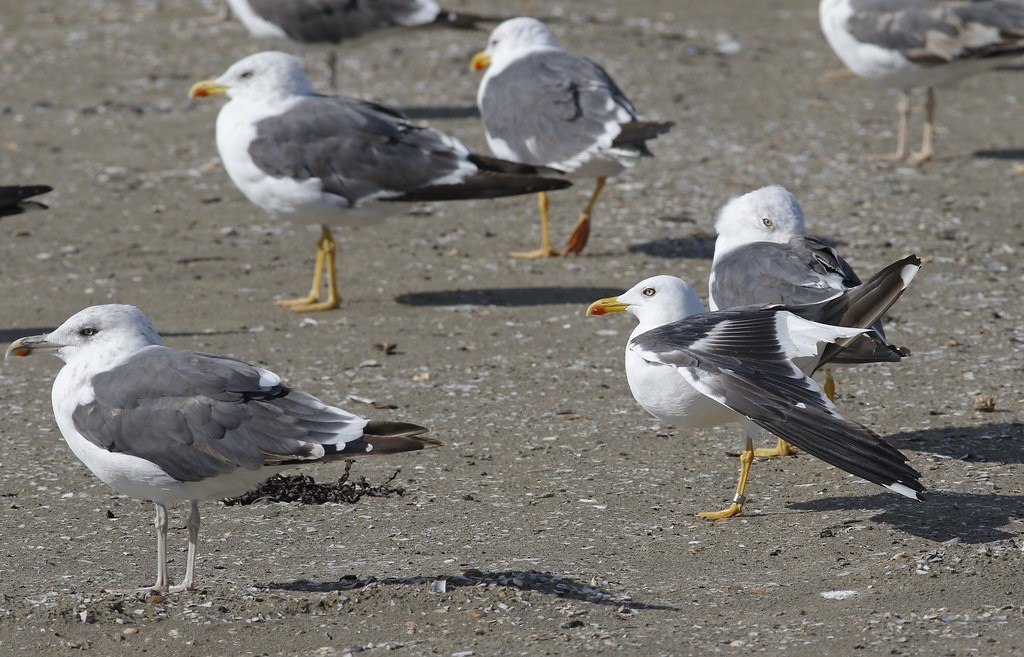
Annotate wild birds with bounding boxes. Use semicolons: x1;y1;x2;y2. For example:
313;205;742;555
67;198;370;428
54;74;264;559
817;1;1024;166
707;184;913;455
470;18;677;257
1;303;443;595
190;50;571;311
585;255;927;521
222;1;480;86
